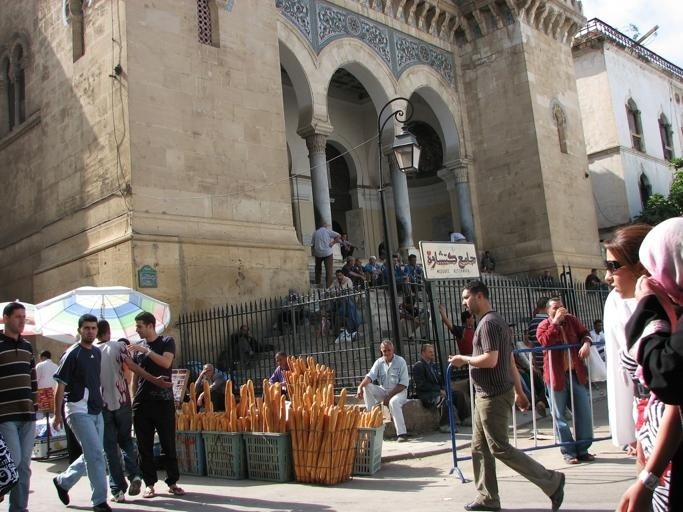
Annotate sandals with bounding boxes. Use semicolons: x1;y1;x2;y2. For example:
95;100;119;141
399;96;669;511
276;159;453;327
168;486;186;496
143;489;155;498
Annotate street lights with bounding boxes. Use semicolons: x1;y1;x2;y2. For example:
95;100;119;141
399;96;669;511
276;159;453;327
378;95;423;357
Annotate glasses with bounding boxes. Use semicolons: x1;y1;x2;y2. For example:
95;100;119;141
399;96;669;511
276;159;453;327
604;260;624;272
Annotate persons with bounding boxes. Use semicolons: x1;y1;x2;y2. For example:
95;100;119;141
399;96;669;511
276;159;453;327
195;223;459;443
440;217;682;512
1;303;184;510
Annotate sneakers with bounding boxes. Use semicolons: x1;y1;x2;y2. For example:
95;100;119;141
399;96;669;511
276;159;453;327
549;473;566;511
397;434;408;443
463;502;501;511
110;490;126;503
52;476;71;506
93;502;112;511
127;478;142;496
537;402;548;418
567;453;595;465
460;419;472;427
439;425;459;433
